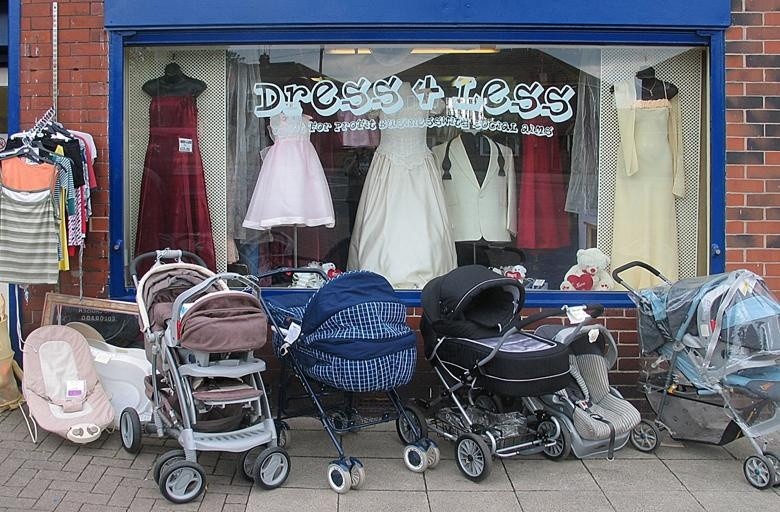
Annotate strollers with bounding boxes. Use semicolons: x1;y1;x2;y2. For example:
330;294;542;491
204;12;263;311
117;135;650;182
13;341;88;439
122;247;292;502
529;318;662;461
611;260;780;489
251;265;439;492
398;261;570;483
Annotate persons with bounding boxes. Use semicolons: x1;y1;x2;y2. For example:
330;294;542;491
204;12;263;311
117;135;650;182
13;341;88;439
133;61;215;277
243;108;335;232
611;70;684;287
345;81;457;290
431;131;520;252
516;92;568;250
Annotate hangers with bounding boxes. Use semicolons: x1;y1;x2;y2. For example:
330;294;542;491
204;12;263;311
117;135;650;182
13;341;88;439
0;110;75;173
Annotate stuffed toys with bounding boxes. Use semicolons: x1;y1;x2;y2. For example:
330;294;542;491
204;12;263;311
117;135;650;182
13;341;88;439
321;262;342;280
562;247;611;294
503;265;526;283
